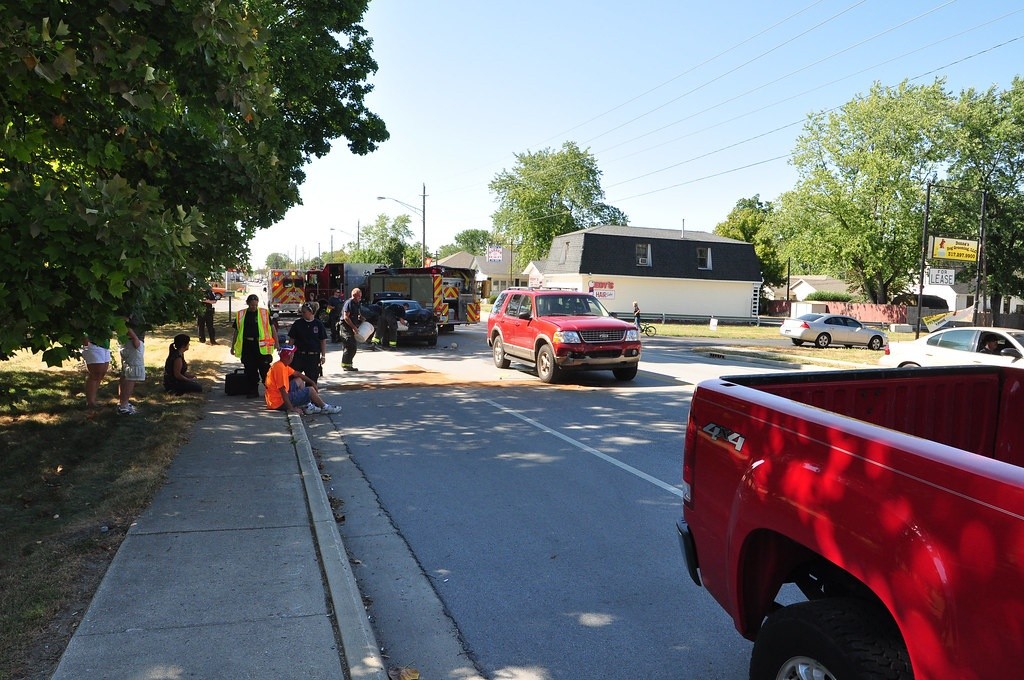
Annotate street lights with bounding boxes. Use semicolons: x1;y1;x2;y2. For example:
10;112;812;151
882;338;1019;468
330;227;360;251
376;196;425;267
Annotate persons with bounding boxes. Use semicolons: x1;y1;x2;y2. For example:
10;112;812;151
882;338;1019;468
230;295;279;398
265;345;342;414
195;284;219;345
163;334;202;393
633;302;642;332
79;332;112;409
117;314;146;415
328;289;342;343
340;288;365;371
371;302;409;351
288;302;328;388
978;333;1001;354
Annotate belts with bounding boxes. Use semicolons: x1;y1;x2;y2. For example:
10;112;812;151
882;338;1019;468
298;350;318;355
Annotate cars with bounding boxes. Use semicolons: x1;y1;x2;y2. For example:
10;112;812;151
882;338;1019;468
878;326;1024;370
369;299;439;347
780;313;887;351
206;281;226;300
247;278;268;292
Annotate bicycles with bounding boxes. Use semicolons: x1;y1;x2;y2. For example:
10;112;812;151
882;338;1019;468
632;315;656;337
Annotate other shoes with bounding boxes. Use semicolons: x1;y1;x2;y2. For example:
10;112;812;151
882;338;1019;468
247;390;259;398
344;365;358;372
116;402;140;415
371;342;376;351
333;338;337;343
338;338;342;342
210;340;218;344
389;346;398;351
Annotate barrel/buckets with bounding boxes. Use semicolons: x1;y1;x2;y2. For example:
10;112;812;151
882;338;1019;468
388;320;409;330
354;322;375;342
710;318;718;330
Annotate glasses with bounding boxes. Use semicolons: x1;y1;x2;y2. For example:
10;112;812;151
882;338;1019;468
335;292;340;293
304;302;314;314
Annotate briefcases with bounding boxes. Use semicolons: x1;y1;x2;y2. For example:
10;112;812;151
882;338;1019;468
225;368;247;395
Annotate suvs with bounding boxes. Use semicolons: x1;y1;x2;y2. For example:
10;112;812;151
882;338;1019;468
487;287;641;382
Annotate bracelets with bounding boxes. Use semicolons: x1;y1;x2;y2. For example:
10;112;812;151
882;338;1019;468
322;356;325;358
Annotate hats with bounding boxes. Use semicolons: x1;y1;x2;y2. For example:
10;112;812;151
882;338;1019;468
278;344;296;357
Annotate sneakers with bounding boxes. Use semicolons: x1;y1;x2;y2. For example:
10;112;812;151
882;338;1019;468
320;404;342;414
305;404;321;415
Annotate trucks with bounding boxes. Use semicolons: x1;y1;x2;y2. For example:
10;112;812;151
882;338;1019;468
263;270;308;316
303;263;483;335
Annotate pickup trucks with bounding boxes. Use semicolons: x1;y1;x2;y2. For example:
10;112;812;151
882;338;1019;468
675;366;1024;680
